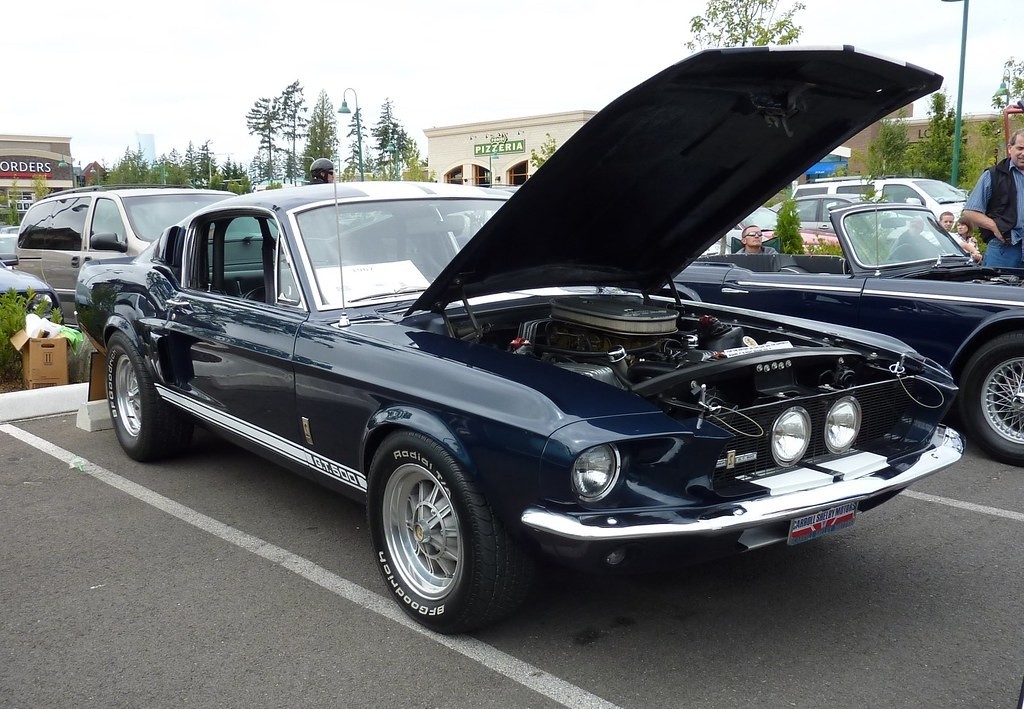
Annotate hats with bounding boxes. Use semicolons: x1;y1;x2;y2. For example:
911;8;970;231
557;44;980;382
909;216;924;230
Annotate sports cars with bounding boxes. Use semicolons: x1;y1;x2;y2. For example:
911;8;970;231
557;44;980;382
618;203;1024;469
72;43;970;639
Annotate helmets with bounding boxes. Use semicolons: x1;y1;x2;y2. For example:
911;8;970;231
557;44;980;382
310;158;333;177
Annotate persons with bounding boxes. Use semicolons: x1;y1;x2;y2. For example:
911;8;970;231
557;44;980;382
735;225;779;254
888;211;983;264
308;158;335;186
963;128;1024;269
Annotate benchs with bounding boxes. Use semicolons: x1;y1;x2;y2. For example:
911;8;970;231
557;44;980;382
208;265;340;304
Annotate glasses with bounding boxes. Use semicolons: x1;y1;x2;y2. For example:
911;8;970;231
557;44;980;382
744;231;761;238
324;171;333;175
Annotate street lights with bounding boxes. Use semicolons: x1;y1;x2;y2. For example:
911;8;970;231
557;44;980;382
337;88;364;181
993;68;1011;161
387;138;400;180
488;143;500;188
58;150;75;188
941;0;970;187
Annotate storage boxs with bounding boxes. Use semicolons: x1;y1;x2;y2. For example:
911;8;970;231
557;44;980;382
10;313;84;390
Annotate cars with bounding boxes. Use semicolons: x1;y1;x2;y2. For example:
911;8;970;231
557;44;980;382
0;174;969;327
